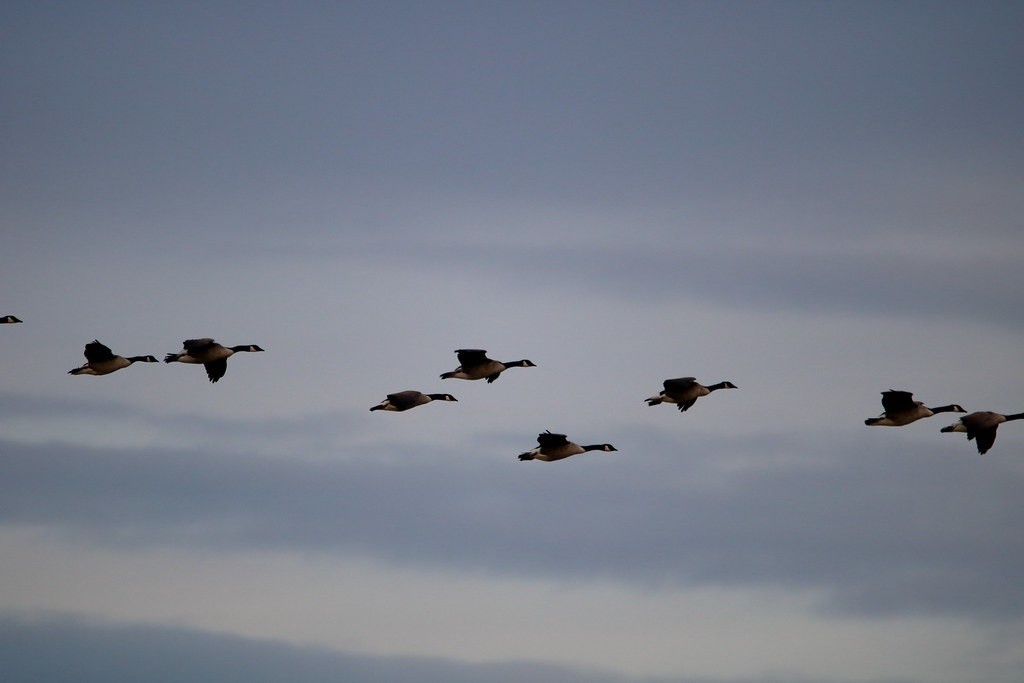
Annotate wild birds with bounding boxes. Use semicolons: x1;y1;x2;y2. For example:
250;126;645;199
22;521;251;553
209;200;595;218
864;389;968;427
644;377;738;412
940;411;1024;455
439;349;537;383
517;430;619;462
66;339;161;376
163;338;265;384
367;390;458;412
